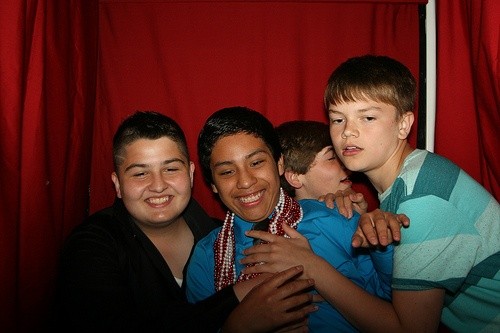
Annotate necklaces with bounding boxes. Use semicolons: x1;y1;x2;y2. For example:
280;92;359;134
214;186;303;293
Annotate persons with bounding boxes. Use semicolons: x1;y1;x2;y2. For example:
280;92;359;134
53;110;319;333
270;118;353;200
237;54;500;333
181;105;411;333
